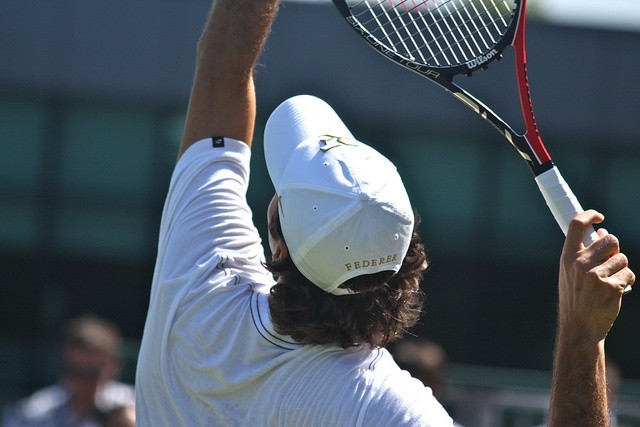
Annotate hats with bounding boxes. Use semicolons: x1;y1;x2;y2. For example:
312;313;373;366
263;95;415;296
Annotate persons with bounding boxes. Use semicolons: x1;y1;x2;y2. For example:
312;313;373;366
0;312;136;427
135;0;636;427
